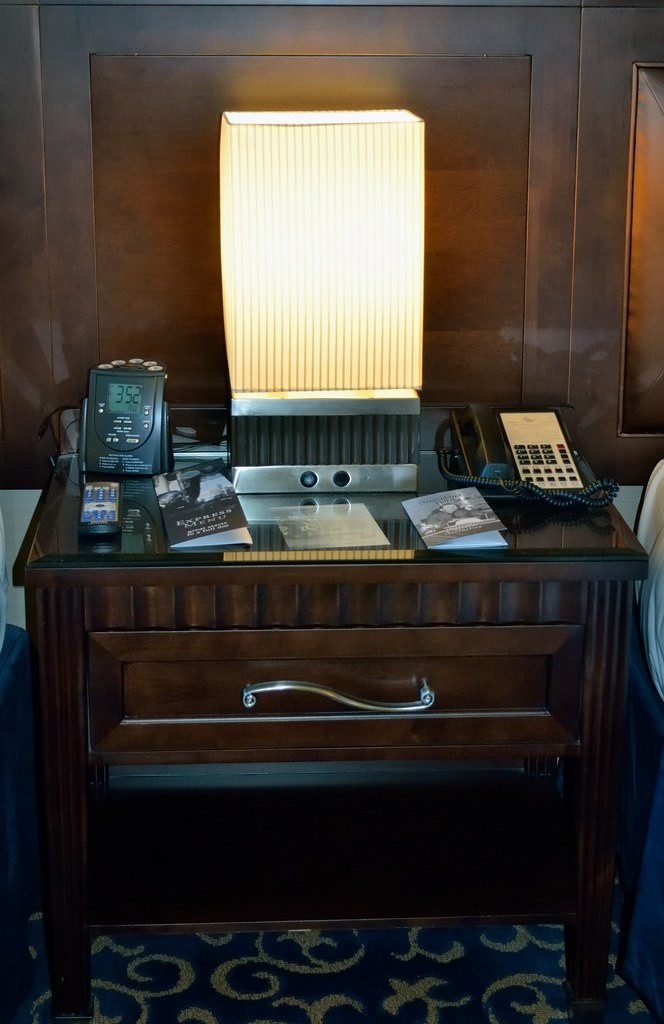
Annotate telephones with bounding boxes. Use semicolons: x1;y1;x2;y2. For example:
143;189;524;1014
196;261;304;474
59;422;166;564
452;405;606;508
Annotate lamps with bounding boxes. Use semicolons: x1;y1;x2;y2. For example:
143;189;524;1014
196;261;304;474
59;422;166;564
220;109;425;493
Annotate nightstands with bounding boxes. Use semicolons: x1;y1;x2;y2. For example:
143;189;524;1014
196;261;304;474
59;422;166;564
12;456;649;1024
